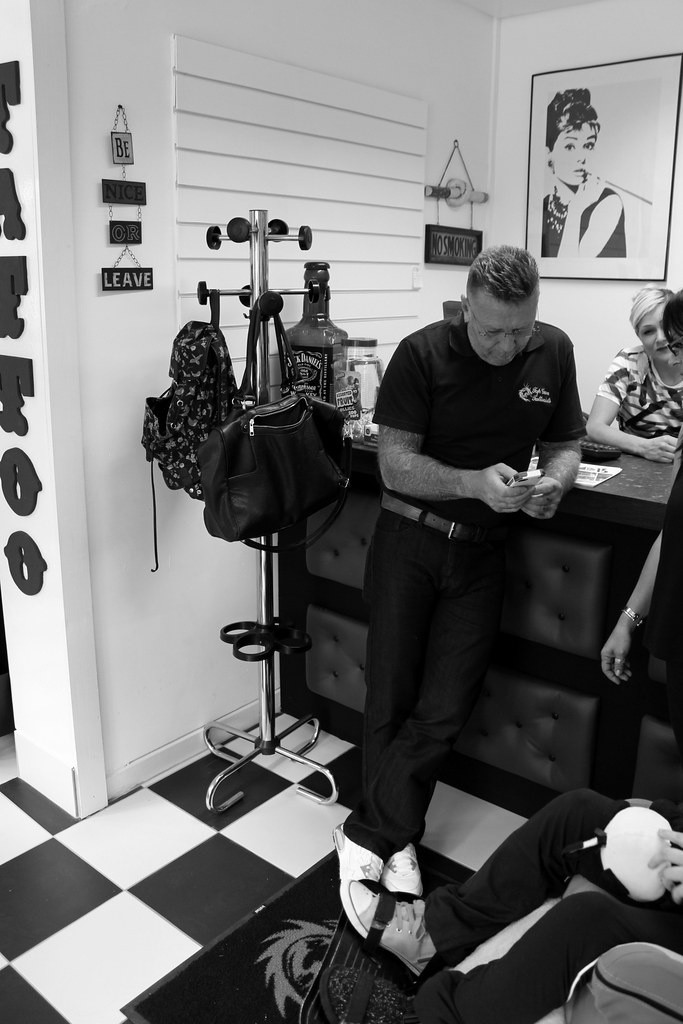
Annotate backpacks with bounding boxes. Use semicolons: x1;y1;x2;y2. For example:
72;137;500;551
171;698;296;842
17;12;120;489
141;289;238;501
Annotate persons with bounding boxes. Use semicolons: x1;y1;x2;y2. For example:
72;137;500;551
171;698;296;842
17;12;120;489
321;787;683;1024
332;247;588;896
603;290;683;749
586;283;683;462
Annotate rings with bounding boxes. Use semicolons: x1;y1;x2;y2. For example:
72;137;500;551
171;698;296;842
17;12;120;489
615;658;625;663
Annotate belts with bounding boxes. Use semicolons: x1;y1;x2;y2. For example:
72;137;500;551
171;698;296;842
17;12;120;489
380;491;489;543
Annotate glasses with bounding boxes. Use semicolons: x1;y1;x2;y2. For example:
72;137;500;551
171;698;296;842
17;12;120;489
667;336;683;356
467;300;541;341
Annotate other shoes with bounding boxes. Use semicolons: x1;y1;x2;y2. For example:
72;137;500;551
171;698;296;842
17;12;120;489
318;965;418;1024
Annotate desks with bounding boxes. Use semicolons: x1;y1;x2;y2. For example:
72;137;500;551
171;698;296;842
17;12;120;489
278;454;683;812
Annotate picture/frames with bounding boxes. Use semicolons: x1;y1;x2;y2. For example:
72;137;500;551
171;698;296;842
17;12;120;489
528;53;683;282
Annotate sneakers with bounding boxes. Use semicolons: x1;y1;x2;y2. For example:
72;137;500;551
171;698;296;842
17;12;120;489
333;823;383;883
381;842;424;896
338;878;437;977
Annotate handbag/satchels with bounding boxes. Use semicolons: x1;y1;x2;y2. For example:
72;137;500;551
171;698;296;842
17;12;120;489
196;297;351;553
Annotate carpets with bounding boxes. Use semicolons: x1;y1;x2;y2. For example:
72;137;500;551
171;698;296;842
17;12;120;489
121;844;477;1024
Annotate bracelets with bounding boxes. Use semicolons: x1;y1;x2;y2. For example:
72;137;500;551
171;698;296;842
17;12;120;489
621;606;643;627
